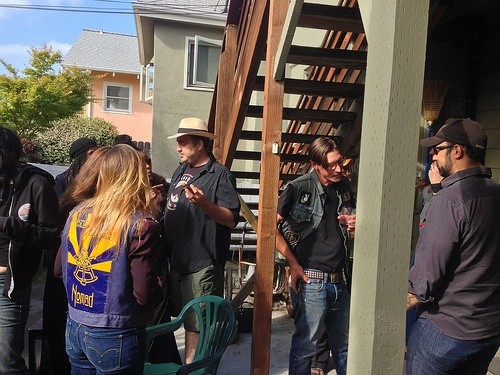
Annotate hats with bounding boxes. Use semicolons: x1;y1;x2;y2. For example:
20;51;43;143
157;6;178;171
0;127;24;167
341;154;351;166
69;137;98;158
420;118;488;149
167;117;217;141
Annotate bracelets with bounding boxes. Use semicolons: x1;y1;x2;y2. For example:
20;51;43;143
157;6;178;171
430;183;444;193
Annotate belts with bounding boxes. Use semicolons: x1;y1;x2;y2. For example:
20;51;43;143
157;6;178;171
303;270;343;283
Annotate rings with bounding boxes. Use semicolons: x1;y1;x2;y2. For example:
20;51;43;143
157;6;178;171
430;169;431;170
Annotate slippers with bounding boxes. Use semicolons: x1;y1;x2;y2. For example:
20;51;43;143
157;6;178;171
311;368;325;375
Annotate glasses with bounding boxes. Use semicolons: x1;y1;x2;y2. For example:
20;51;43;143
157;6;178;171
145;158;152;166
433;145;455;155
327;157;346;170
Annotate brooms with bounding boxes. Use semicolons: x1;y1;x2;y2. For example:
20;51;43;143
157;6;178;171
423;80;448;184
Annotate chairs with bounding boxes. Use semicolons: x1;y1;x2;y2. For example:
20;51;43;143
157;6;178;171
143;295;236;375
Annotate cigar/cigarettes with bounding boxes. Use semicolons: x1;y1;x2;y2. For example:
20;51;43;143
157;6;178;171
181;182;195;196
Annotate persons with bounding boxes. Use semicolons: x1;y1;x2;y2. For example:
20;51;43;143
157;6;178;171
43;135;171;232
418;148;445;233
39;146;113;375
275;136;356;375
164;117;241;364
55;144;166;375
311;159;355;375
0;126;54;375
406;118;500;375
